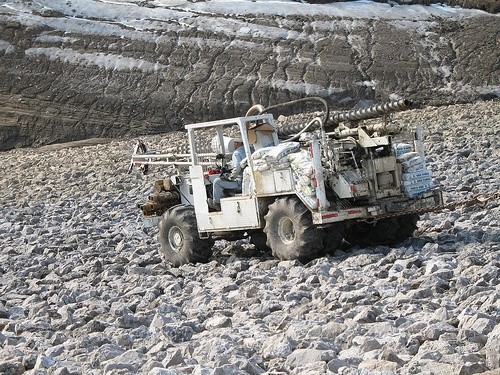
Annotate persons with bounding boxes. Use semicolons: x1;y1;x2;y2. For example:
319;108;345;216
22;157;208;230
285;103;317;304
206;136;253;209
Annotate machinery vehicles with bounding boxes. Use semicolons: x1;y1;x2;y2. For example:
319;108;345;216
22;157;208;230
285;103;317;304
126;114;444;269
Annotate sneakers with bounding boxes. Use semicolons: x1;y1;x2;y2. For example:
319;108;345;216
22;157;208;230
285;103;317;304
208;198;220;210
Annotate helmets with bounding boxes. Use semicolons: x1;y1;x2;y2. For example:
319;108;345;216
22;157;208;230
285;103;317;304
233;132;243;142
